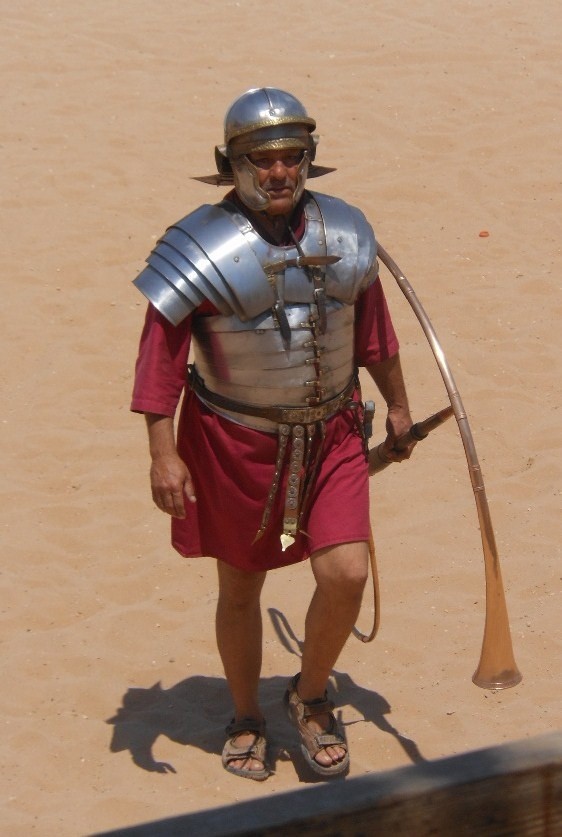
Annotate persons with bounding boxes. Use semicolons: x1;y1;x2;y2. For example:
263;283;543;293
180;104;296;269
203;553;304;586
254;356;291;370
134;85;419;779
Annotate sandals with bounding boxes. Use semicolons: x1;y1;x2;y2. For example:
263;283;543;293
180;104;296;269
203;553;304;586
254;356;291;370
222;717;271;781
283;672;350;776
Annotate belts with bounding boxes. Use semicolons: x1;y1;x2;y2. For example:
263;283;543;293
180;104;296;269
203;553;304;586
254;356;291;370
186;363;359;422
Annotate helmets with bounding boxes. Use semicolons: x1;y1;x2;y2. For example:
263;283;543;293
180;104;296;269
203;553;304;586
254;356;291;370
189;87;338;211
